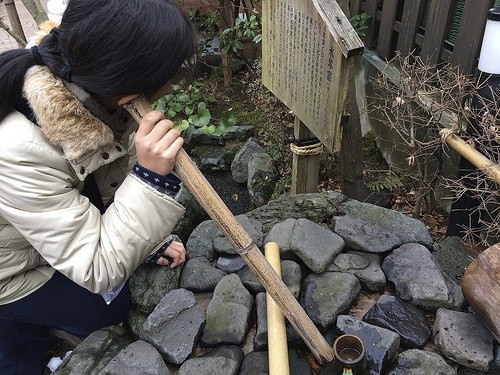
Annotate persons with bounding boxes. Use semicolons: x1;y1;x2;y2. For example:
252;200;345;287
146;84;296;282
0;1;194;347
